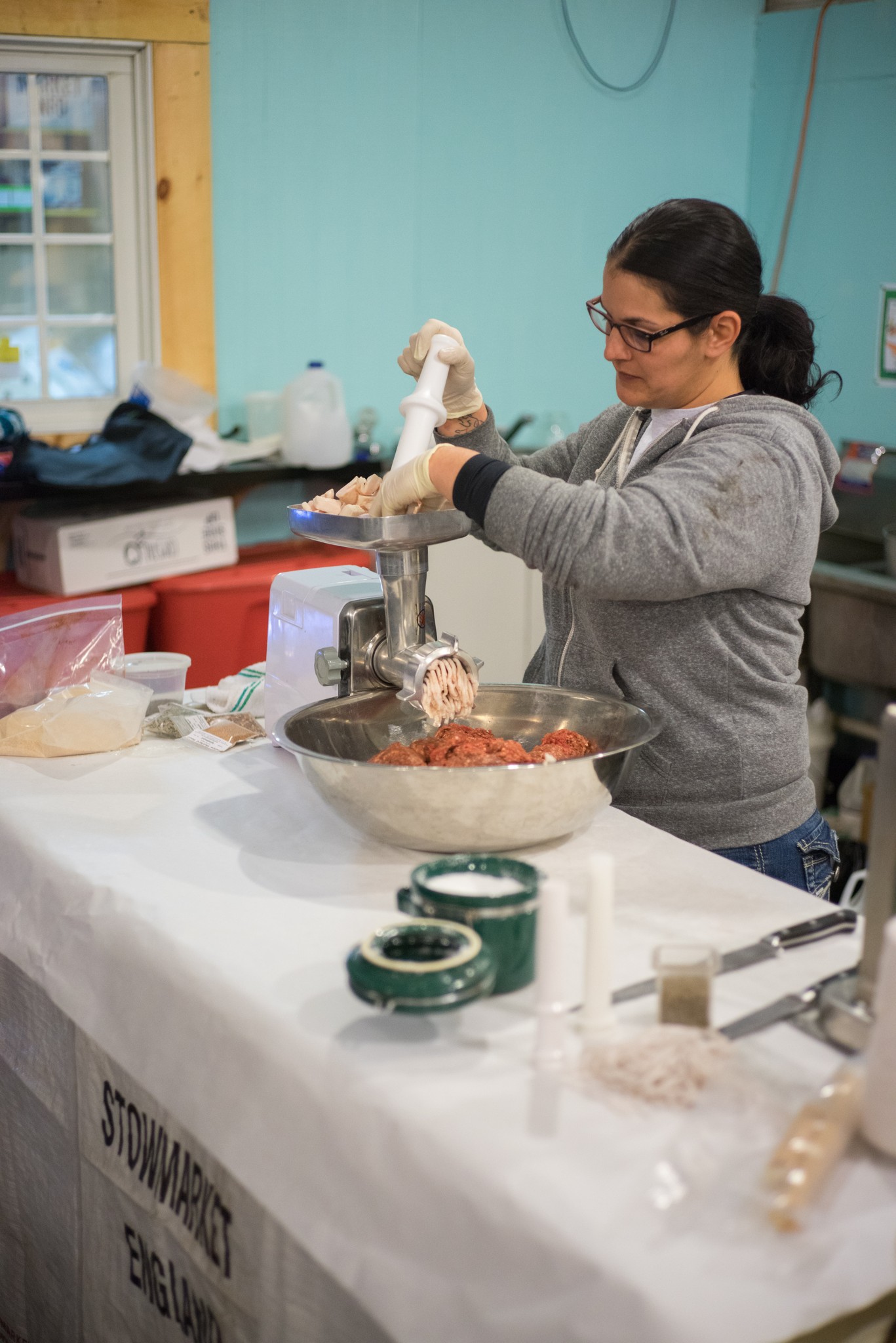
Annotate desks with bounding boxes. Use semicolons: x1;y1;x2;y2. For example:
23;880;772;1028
1;680;894;1342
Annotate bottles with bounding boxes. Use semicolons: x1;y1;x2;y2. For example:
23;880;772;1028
654;935;712;1030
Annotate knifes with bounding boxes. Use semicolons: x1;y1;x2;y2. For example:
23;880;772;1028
718;967;862;1040
564;908;861;1014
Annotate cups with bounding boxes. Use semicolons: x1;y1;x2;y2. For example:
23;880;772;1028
395;858;540;999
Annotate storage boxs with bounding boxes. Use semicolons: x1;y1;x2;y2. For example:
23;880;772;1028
1;490;376;716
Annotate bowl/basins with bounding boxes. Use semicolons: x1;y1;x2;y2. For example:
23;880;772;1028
114;648;191;714
268;677;665;856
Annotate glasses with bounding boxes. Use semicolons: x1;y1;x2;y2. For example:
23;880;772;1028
585;294;719;353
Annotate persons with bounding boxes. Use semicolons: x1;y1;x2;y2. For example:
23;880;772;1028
372;198;842;903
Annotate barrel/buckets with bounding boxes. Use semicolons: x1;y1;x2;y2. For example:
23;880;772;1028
281;357;354;472
